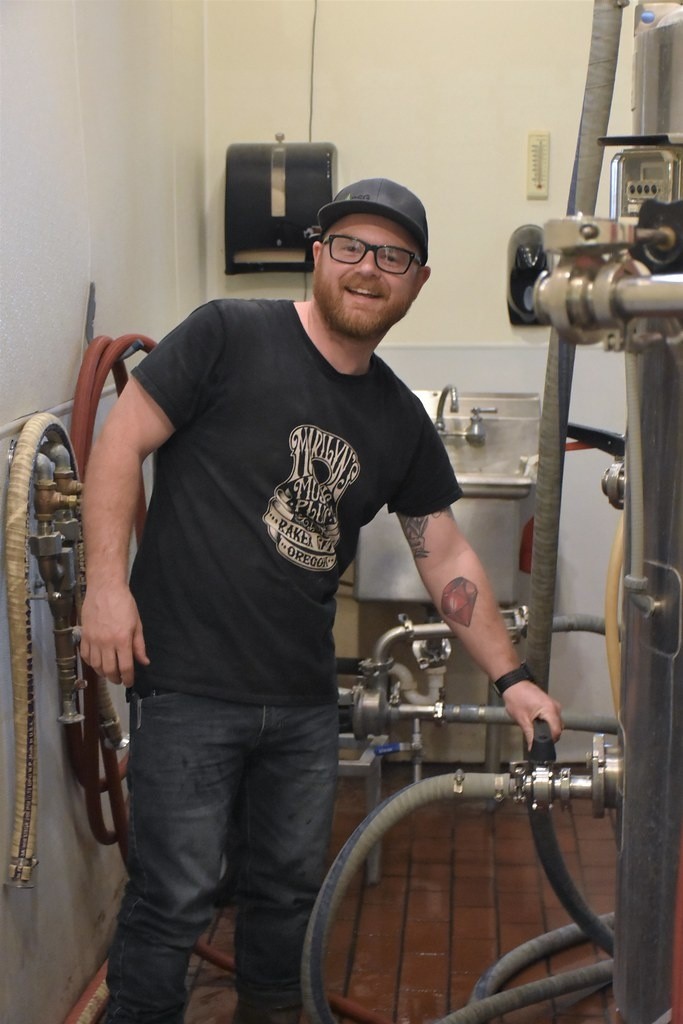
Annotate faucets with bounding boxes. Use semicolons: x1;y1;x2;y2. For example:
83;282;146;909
433;383;499;448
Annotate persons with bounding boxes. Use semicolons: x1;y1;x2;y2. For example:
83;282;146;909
81;178;562;1024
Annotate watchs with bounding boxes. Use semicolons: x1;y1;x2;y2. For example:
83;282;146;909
493;662;534;697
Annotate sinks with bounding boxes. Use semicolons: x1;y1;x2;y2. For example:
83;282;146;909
429;414;541;485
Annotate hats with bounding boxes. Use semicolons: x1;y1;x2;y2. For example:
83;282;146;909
316;178;428;266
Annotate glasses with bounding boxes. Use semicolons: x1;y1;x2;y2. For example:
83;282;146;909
322;234;422;274
